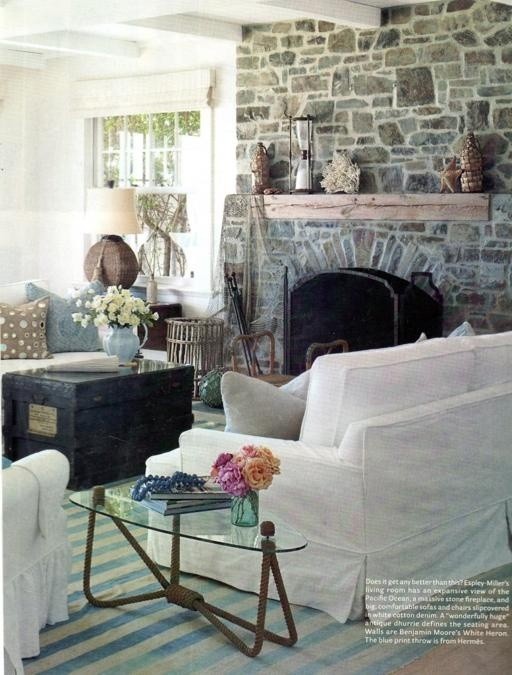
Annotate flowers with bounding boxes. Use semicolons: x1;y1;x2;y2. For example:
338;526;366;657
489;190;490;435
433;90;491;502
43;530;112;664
209;444;284;498
71;283;161;331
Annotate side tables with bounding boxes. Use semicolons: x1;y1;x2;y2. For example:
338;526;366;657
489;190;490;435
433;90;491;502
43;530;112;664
69;473;309;656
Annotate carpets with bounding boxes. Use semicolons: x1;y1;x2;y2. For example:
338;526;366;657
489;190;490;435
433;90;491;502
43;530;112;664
22;402;512;675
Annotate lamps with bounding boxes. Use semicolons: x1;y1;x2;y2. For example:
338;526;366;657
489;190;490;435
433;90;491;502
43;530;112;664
82;180;145;294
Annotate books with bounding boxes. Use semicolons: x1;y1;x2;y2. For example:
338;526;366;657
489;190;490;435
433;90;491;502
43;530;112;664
136;471;233;517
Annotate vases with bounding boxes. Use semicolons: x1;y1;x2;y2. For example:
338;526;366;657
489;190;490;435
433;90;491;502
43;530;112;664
229;493;259;528
146;281;157;303
104;327;149;365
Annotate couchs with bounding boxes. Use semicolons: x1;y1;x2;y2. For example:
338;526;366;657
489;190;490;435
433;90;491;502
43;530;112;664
0;277;125;373
2;447;71;675
146;330;511;623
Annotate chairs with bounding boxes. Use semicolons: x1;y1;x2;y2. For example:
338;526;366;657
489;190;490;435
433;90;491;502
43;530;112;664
306;339;348;371
230;332;297;388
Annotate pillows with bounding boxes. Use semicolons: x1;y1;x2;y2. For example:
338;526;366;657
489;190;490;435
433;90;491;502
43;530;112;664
27;280;102;352
222;372;306;441
0;296;55;358
447;321;477;338
281;370;310;400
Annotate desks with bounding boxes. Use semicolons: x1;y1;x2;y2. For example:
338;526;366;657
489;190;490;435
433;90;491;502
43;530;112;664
137;302;182;350
